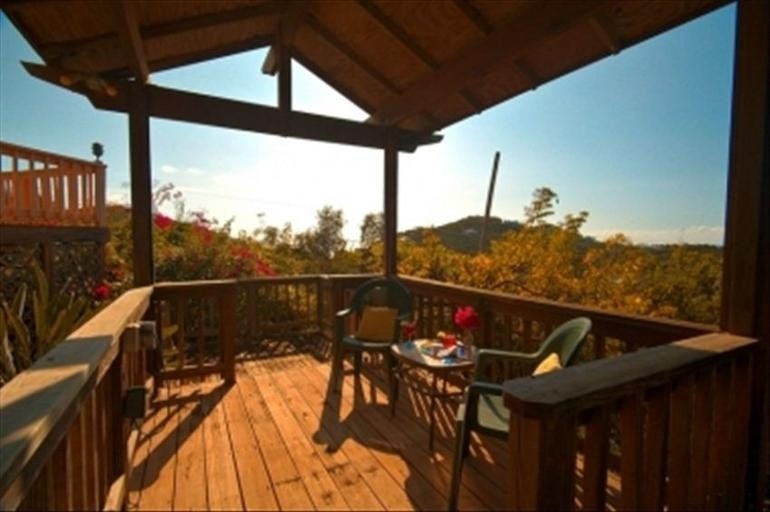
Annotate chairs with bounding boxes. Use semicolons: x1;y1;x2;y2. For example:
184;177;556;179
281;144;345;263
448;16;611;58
334;278;413;413
448;317;593;512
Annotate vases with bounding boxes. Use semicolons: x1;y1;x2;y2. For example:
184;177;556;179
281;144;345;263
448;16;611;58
463;330;473;361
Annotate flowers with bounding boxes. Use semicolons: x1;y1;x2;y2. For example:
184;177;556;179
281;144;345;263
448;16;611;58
453;305;480;330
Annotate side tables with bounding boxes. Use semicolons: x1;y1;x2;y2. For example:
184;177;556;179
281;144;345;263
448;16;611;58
390;339;476;453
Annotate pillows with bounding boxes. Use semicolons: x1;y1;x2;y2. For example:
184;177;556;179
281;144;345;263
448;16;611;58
531;352;562;376
355;305;399;341
